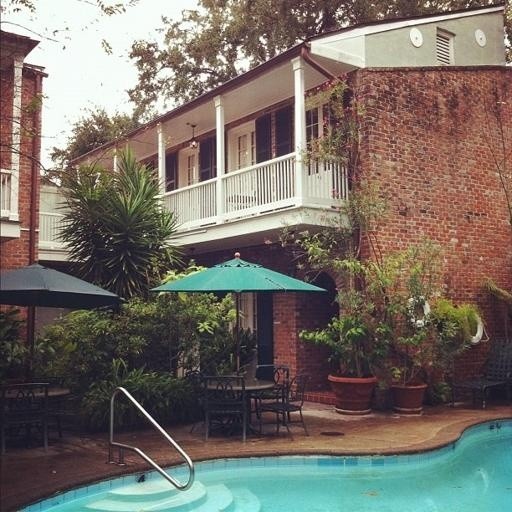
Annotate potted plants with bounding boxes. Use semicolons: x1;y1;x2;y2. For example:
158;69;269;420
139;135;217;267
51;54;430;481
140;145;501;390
296;286;379;416
254;174;463;415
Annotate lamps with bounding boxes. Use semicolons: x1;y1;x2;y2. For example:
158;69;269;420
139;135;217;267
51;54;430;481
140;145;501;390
188;124;199;148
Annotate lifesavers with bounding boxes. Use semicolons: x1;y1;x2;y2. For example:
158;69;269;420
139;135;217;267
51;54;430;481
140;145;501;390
462;311;485;345
407;296;431;328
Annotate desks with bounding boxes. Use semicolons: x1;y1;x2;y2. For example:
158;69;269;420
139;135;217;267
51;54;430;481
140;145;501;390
1;386;72;441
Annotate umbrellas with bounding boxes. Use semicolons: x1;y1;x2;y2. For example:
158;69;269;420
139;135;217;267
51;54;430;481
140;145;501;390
0;258;129;391
149;251;329;386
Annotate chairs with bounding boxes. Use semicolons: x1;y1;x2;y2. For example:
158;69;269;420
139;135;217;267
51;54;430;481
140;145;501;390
444;339;512;412
37;369;65;439
183;361;311;445
1;381;52;454
